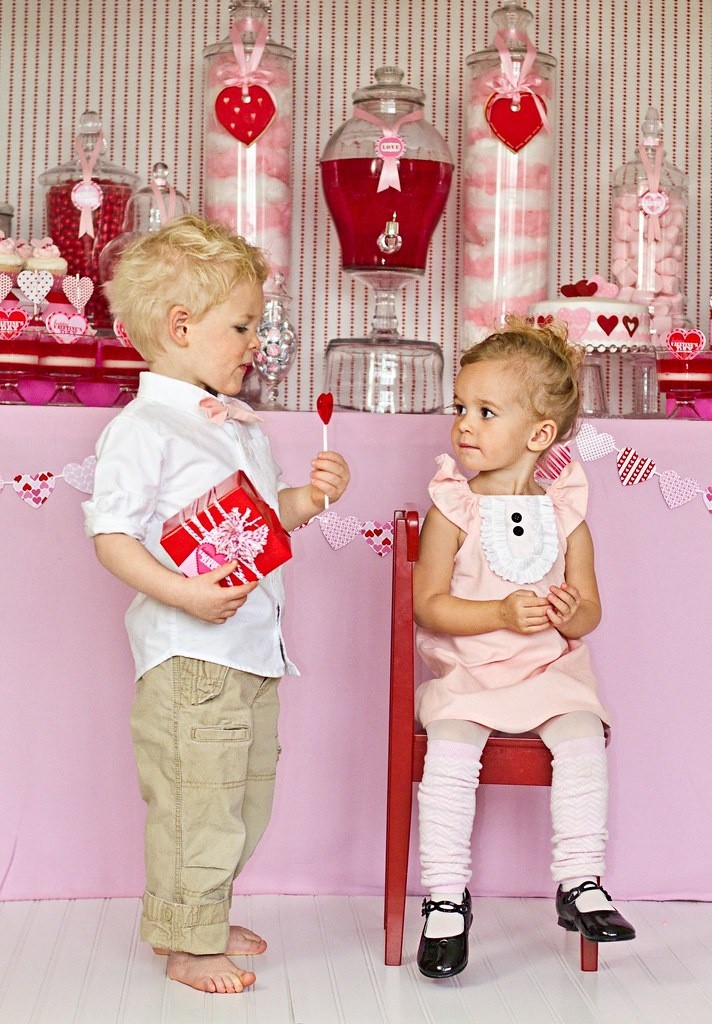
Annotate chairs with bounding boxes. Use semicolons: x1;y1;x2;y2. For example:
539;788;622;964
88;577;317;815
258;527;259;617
383;510;612;972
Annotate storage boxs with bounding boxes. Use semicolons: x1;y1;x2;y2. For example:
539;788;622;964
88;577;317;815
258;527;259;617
160;470;292;587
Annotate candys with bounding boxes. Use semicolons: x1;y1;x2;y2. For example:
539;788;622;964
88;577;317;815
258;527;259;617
317;392;334;425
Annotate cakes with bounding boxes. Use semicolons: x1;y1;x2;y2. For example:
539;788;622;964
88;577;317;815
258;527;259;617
522;273;651;347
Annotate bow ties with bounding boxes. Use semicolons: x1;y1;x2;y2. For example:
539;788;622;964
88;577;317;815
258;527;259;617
201;398;264;427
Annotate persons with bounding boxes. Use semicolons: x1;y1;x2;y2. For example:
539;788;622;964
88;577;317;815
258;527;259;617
409;319;640;980
82;214;354;998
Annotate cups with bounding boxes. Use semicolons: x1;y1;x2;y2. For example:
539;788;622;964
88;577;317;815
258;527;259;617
656;350;712;421
0;331;38;404
98;336;149;406
39;332;98;404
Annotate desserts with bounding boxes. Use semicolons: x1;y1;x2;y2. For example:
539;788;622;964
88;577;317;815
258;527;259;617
0;229;68;277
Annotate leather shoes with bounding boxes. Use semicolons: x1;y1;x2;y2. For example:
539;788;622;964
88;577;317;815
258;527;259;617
417;887;473;979
557;883;636;942
